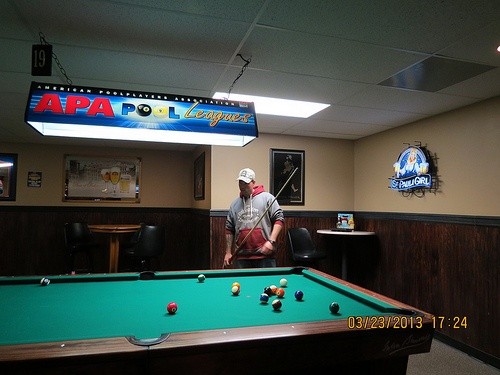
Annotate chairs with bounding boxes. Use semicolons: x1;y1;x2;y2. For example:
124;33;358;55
63;222;96;273
287;227;319;268
125;226;164;272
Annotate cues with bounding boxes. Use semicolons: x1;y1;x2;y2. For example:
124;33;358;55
224;167;298;270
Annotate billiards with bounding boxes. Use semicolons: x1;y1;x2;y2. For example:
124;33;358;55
295;290;303;299
198;274;205;282
280;279;287;287
168;303;177;314
272;300;282;310
329;302;339;313
41;278;50;285
259;285;284;303
232;282;240;295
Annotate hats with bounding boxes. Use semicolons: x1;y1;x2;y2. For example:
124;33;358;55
236;168;255;184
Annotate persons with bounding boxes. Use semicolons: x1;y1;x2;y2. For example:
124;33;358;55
221;168;285;269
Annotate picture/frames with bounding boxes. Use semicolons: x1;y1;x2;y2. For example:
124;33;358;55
193;151;206;200
62;154;143;203
0;152;19;202
269;148;305;206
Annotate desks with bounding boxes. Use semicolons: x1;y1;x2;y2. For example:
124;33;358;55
88;223;142;273
0;267;436;375
317;228;375;281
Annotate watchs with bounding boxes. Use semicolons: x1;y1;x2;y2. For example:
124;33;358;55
268;239;276;245
